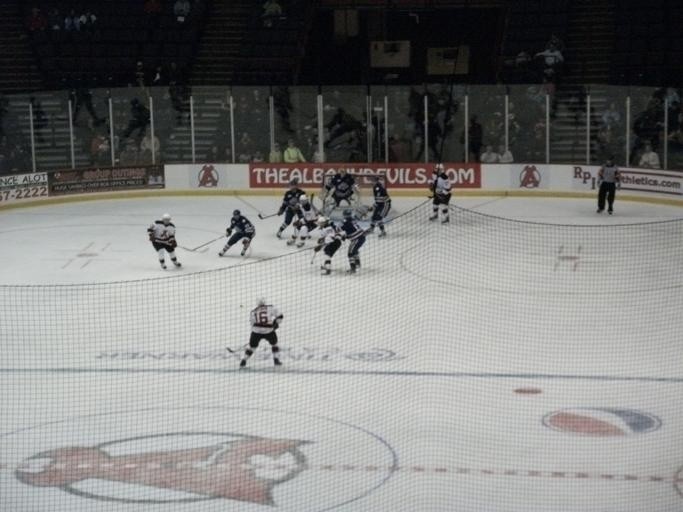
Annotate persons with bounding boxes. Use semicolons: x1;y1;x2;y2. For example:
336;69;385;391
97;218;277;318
428;161;452;224
593;154;622;216
275;166;392;277
0;0;683;171
239;295;284;370
215;208;255;258
144;213;182;271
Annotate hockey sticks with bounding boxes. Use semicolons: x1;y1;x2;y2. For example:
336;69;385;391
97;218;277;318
156;235;226;253
258;212;276;219
226;343;248;353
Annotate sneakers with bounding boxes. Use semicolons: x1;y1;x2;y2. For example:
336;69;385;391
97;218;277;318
609;211;612;215
240;359;247;366
161;264;167;270
430;214;438;220
241;249;245;256
275;358;281;365
378;233;386;237
366;227;374;232
172;261;181;266
596;209;603;213
218;249;224;256
442;218;449;223
321;259;332;273
346;258;360;272
277;229;304;247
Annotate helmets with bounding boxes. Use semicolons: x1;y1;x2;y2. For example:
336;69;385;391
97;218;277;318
162;213;170;218
233;210;240;215
256;300;264;306
437;165;444;169
290;168;353;224
